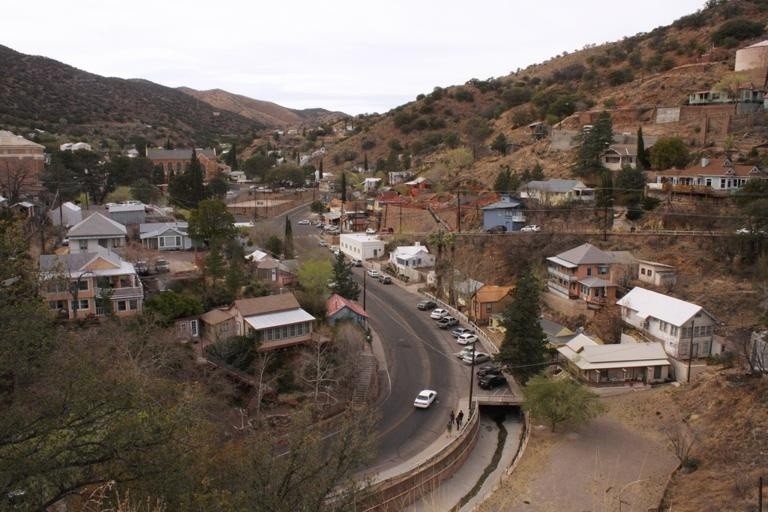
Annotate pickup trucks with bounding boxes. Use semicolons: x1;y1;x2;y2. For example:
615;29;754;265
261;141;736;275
437;317;460;329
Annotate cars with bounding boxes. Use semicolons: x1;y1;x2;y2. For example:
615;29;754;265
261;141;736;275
297;220;345;257
457;346;475;359
463;352;491;365
155;259;170;272
103;201;154;212
477;366;497;376
248;185;309;194
134;260;149;273
736;227;766;235
431;308;449;319
367;270;378;278
414;389;437;408
366;228;376;235
451;328;475;336
417;300;436;310
479;375;507;389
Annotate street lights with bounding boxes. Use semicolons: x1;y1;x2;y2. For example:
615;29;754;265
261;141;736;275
604;197;615;240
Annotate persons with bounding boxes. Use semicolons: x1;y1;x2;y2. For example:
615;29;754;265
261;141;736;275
450;410;454;424
459;409;464;426
456;415;461;430
446;421;452;438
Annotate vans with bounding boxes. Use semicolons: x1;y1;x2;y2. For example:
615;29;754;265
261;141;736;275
378;274;391;284
379;227;394;235
350;258;362;266
520;225;542;232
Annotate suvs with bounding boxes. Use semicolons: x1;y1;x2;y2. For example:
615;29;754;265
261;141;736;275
487;224;507;234
457;334;478;345
